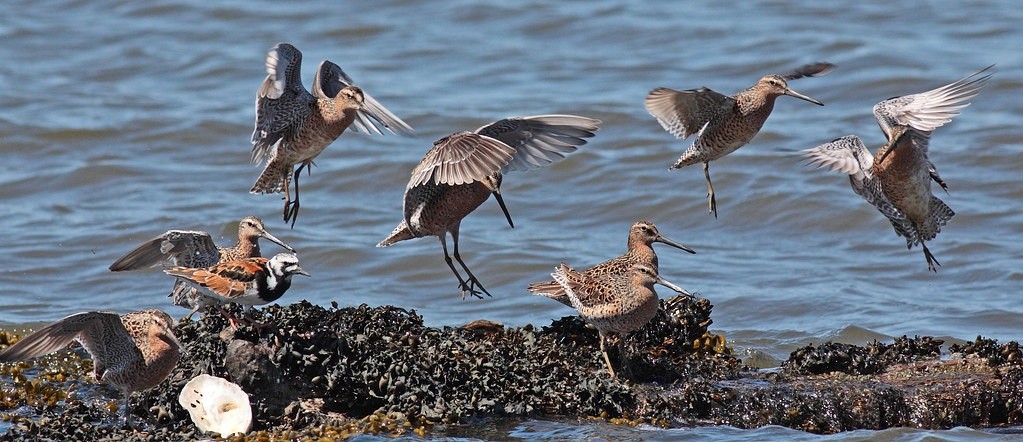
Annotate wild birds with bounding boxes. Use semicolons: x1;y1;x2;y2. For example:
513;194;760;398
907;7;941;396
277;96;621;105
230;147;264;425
108;215;312;334
643;61;839;221
792;62;1000;274
526;219;698;383
249;42;419;231
374;113;603;301
0;308;189;434
